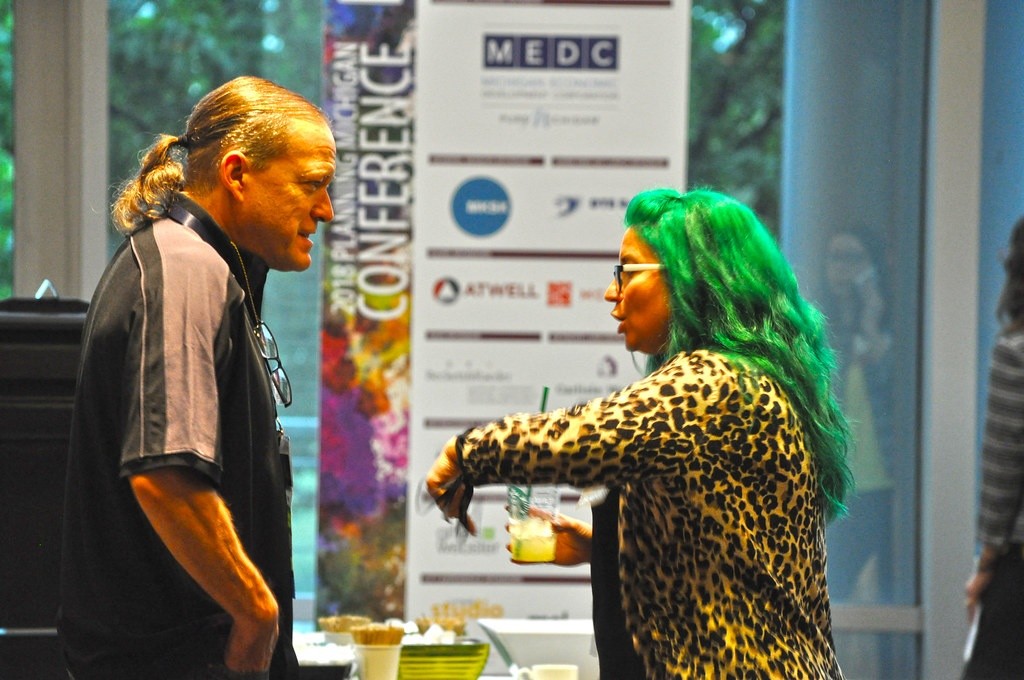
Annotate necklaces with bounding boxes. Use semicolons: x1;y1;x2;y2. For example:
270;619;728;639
232;242;259;324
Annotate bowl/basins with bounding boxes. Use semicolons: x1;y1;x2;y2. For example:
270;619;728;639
399;635;489;680
476;615;600;680
298;664;352;680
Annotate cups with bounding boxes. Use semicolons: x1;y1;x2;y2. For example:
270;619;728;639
353;644;400;680
518;664;579;680
508;484;557;562
324;632;353;645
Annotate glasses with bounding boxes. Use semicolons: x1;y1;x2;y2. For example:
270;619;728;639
252;319;293;410
610;262;666;295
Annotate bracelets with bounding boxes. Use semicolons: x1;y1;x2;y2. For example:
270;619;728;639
456;425;473;477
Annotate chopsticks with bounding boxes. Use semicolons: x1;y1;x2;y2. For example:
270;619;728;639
319;614;404;645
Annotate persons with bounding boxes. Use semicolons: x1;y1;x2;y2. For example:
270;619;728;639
425;188;859;680
961;216;1024;680
802;219;894;680
58;76;333;680
